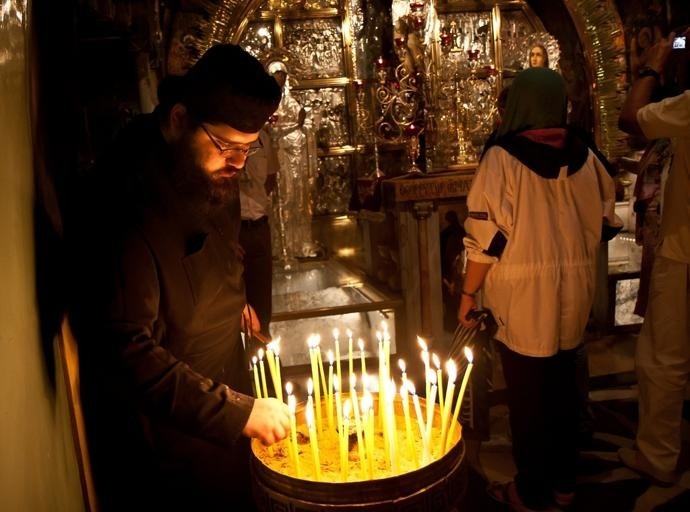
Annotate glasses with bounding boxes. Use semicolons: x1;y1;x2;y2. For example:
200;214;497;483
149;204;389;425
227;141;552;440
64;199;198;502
201;125;264;159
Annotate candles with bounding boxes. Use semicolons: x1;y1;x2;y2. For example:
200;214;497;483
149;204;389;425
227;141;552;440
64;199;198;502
253;342;473;483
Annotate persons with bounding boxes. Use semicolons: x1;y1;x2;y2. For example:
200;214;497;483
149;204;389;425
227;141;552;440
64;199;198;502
65;46;292;512
456;24;690;512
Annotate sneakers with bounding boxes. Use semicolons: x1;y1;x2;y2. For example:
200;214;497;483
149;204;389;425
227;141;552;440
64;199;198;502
486;478;579;511
618;444;677;488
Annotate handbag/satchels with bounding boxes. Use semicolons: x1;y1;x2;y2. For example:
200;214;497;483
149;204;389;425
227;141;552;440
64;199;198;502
442;308;501;431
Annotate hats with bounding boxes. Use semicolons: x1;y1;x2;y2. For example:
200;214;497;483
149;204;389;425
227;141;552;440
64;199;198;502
171;43;282;133
